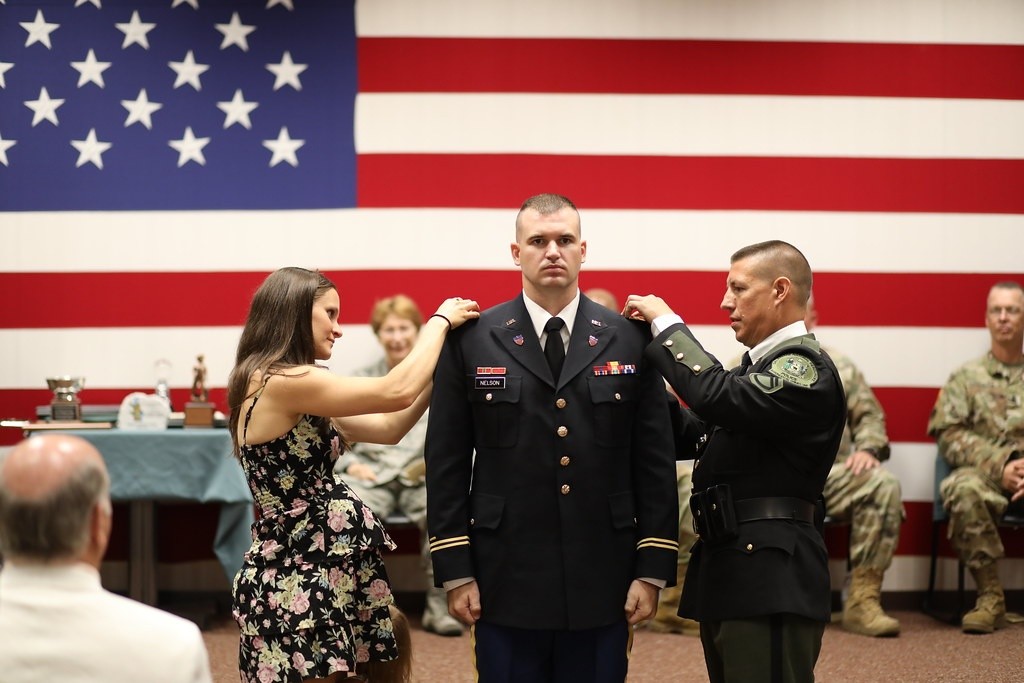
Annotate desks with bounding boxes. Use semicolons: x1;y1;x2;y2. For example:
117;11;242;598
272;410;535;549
24;426;232;603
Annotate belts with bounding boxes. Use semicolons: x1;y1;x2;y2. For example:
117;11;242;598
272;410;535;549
733;497;816;524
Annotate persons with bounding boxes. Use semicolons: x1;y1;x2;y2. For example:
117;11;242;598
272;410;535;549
230;240;906;683
927;282;1024;634
422;192;680;683
0;434;213;682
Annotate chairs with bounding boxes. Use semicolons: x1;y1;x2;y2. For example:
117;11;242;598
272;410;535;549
921;449;1024;628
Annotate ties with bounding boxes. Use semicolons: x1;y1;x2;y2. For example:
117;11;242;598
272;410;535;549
543;317;565;386
737;351;752;376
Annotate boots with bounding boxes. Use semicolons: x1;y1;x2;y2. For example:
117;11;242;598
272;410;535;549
843;569;900;637
420;579;464;635
647;578;700;636
961;561;1007;632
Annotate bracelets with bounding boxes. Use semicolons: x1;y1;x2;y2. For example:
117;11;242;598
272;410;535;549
430;314;451;332
